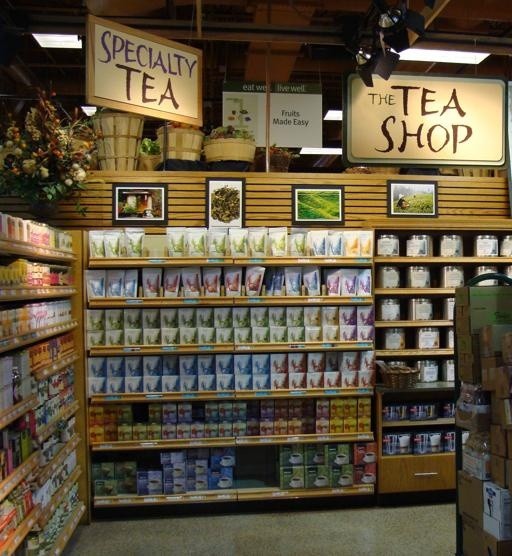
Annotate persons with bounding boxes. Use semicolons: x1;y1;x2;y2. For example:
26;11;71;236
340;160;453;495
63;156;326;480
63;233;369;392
215;191;224;208
396;194;410;210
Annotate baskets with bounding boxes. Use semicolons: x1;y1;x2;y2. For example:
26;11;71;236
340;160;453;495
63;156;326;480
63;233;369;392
138;153;161;171
157;128;256;164
91;112;145;172
379;367;420;388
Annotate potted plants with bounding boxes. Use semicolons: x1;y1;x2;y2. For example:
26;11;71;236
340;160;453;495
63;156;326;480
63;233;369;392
138;121;302;175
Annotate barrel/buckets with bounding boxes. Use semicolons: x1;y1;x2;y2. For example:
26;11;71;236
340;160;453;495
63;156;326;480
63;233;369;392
157;126;205;161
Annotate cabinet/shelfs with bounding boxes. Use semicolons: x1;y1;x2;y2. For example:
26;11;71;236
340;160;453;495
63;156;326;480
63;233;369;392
373;225;511;502
86;225;375;522
454;273;511;555
0;214;91;556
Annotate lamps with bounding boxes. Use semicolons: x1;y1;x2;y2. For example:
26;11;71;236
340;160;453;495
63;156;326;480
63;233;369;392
344;0;430;89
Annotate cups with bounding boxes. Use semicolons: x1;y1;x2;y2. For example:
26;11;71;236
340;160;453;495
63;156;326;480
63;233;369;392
384;432;455;454
145;477;163;490
288;451;379;488
171;455;236;494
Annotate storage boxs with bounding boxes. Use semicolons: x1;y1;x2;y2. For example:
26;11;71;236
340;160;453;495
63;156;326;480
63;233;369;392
455;285;511;555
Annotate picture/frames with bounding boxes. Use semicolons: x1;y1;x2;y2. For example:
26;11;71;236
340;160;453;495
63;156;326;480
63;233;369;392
111;182;170;225
387;180;439;219
204;175;246;231
290;183;345;226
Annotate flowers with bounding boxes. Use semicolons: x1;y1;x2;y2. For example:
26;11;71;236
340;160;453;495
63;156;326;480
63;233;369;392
0;87;105;219
